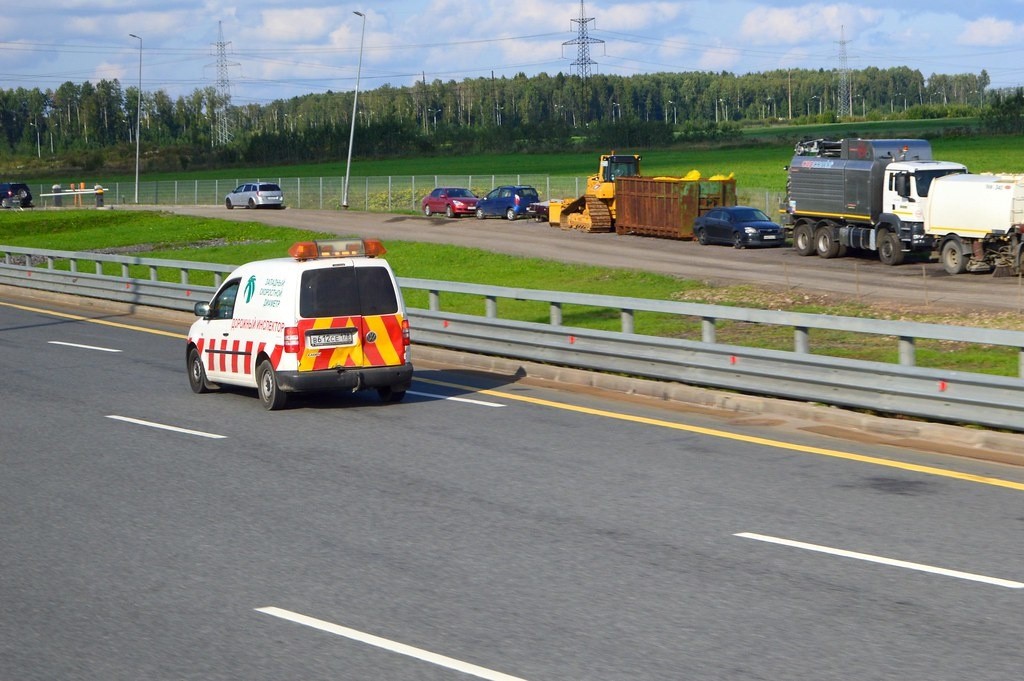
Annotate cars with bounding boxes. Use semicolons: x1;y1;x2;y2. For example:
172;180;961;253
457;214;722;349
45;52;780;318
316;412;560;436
421;187;480;218
692;205;786;249
475;184;540;222
184;237;415;412
224;181;284;210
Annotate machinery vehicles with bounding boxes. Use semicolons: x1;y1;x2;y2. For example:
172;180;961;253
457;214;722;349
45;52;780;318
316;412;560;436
549;151;645;234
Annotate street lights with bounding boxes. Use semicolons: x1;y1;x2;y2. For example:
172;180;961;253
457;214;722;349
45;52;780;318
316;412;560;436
337;10;367;206
129;33;144;208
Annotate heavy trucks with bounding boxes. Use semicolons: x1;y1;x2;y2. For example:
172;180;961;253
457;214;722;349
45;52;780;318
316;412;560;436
781;136;970;267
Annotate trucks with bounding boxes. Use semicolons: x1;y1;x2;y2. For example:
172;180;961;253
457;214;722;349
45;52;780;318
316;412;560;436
924;171;1024;276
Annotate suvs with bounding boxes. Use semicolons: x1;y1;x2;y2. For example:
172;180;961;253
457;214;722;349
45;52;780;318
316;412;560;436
0;182;33;209
